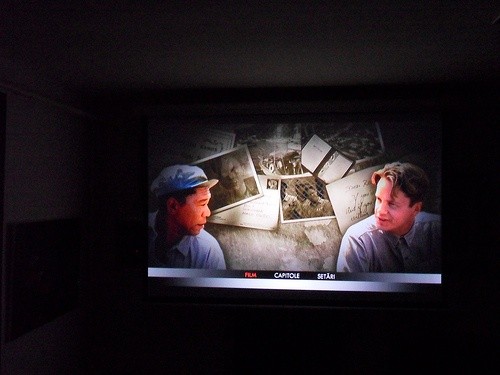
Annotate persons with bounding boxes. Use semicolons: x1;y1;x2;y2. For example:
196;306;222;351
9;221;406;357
215;155;259;210
336;162;441;274
280;180;335;222
148;164;226;271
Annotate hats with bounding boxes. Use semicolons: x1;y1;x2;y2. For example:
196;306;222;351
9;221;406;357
157;164;218;194
